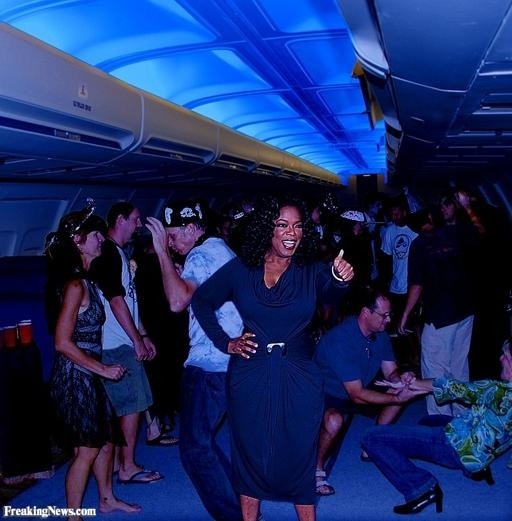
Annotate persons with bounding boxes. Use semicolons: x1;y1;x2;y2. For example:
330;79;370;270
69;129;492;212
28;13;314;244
44;181;511;521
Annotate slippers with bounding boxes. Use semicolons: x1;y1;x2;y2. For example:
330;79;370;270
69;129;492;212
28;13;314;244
146;432;180;445
113;464;143;474
117;469;165;483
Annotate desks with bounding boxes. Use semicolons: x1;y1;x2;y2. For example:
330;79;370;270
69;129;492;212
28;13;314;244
0;341;56;486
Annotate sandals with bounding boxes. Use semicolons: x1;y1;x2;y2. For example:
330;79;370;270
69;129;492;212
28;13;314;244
315;470;335;495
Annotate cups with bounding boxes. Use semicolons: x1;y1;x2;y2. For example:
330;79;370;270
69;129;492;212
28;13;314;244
0;318;32;349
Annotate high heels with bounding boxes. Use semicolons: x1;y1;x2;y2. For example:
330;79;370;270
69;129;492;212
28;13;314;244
393;483;443;513
471;465;494;485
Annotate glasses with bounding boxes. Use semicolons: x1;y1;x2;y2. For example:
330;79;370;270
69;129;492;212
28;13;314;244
373;310;394;319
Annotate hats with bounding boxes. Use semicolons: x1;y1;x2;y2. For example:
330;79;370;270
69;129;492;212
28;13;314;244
161;200;203;227
